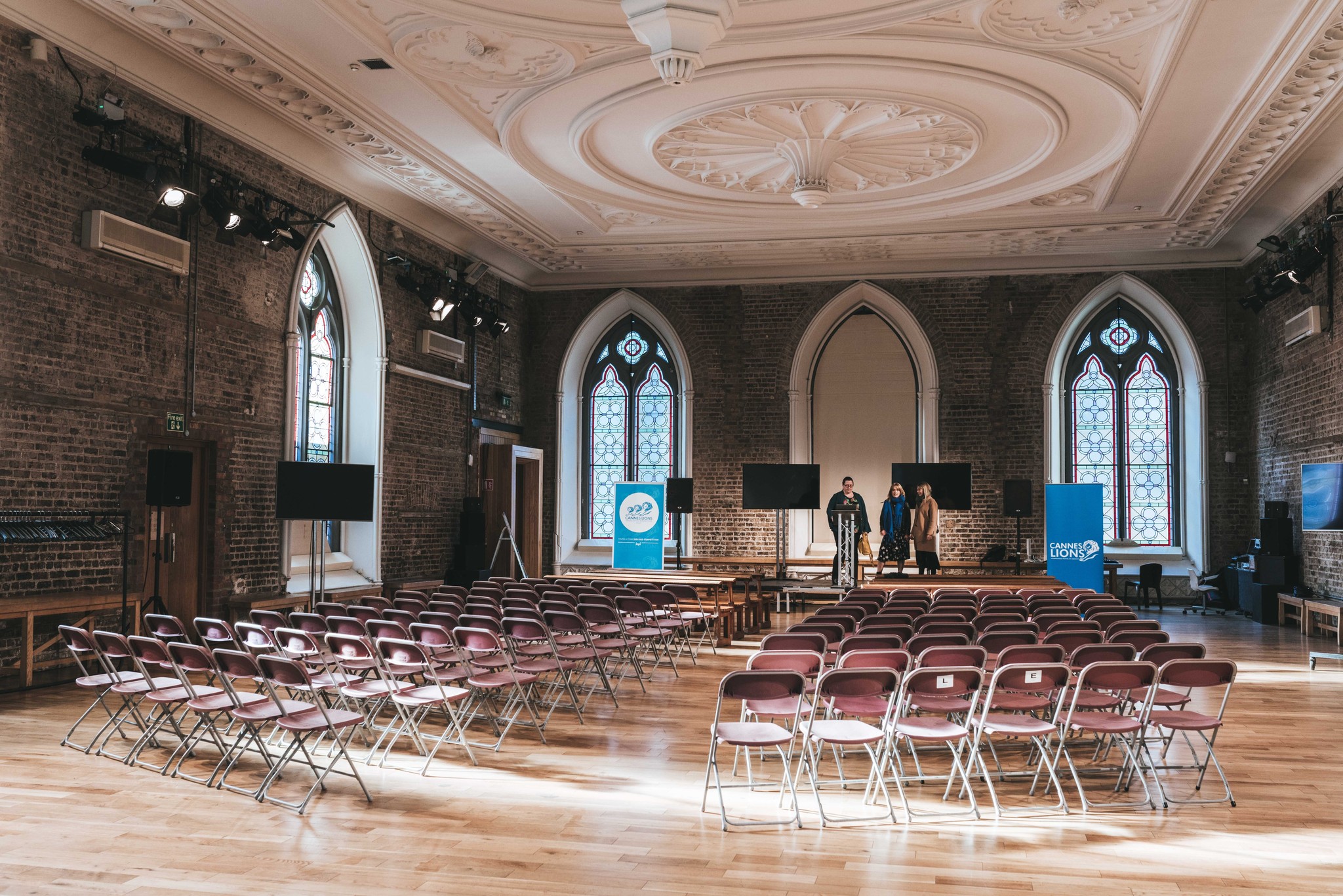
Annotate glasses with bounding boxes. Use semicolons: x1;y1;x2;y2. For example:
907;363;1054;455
843;484;854;488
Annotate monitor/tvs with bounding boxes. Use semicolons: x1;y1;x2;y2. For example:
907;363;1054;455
1301;462;1343;532
892;462;972;510
274;461;375;522
743;463;820;510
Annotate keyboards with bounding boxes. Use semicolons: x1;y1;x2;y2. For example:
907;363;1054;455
1104;559;1119;563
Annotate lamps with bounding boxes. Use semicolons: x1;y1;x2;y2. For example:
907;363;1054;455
65;95;514;340
1238;207;1343;317
20;38;48;66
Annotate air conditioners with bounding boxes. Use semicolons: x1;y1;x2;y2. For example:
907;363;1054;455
82;208;191;279
416;330;465;364
1284;304;1328;350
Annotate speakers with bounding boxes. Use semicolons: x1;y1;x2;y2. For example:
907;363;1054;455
1003;479;1033;516
145;450;193;507
1257;501;1299;586
666;478;693;514
444;497;493;591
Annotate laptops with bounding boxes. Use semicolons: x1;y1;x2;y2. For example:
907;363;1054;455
836;504;857;511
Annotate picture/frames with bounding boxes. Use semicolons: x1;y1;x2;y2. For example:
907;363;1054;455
1301;463;1343;532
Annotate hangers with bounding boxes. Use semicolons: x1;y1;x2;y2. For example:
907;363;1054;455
0;509;136;544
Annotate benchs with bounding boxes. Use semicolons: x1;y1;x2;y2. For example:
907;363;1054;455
1277;592;1327;635
315;584;383;604
1304;600;1343;647
226;593;311;632
544;568;774;649
384;578;444;604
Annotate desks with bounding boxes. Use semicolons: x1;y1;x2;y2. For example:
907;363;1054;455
0;590;143;688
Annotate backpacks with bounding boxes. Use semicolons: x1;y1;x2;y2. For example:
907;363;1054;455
980;544;1007;573
858;532;875;568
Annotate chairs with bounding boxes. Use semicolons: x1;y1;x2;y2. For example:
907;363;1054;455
57;577;1236;814
1182;569;1226;616
1123;562;1163;611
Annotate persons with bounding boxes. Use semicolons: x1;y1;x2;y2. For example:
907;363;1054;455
877;483;911;575
827;477;872;585
909;481;941;575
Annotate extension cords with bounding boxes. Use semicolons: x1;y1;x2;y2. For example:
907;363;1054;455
1204;575;1219;580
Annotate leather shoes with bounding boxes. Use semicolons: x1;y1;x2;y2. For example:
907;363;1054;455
832;579;843;585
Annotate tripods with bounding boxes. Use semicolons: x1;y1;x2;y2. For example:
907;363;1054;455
120;507;169;635
